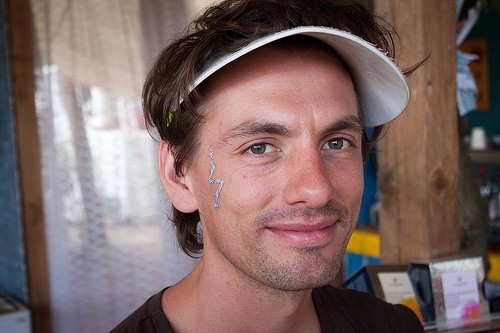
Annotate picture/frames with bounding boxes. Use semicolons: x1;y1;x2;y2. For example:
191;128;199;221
365;266;422;323
427;256;489;323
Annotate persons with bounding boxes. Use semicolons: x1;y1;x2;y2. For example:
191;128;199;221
109;0;426;332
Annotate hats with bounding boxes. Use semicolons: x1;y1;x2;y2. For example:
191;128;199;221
165;22;411;129
